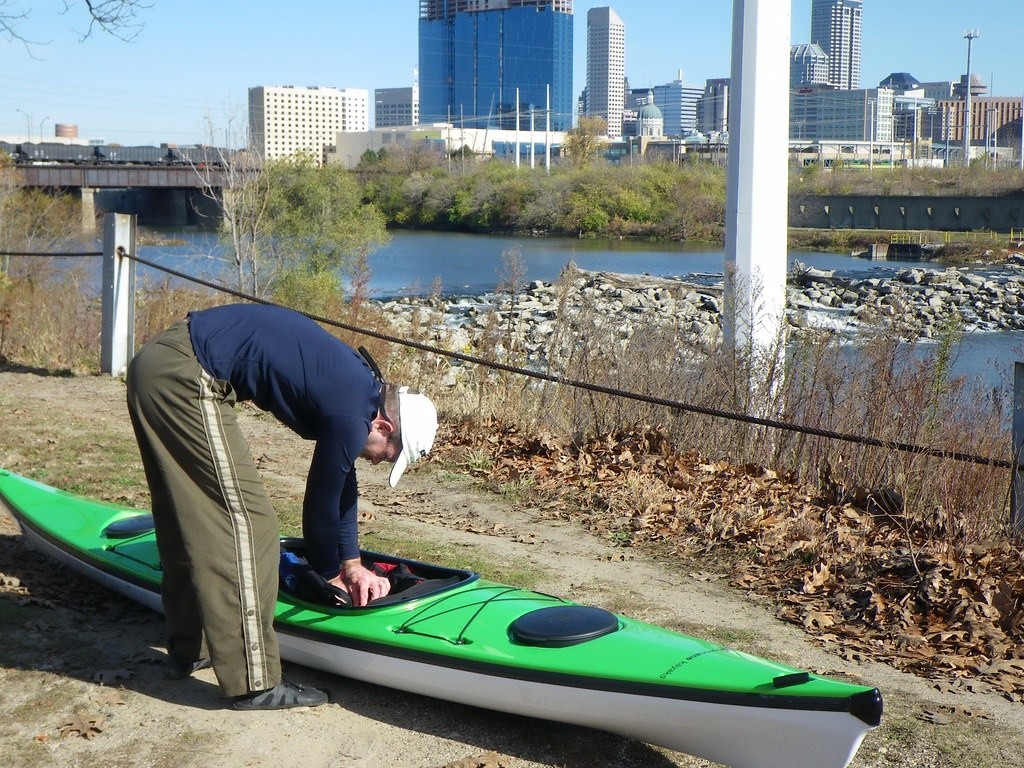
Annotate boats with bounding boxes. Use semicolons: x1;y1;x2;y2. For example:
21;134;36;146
0;470;885;768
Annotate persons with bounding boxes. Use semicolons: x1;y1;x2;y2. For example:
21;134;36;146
126;303;439;711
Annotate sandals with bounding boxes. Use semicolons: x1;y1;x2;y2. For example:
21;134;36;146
189;659;212;672
232;673;329;711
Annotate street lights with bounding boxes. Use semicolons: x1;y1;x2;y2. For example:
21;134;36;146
16;108;31;156
39;117;51;152
963;28;980;169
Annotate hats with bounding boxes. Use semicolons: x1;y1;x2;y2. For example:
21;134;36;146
389;385;438;489
